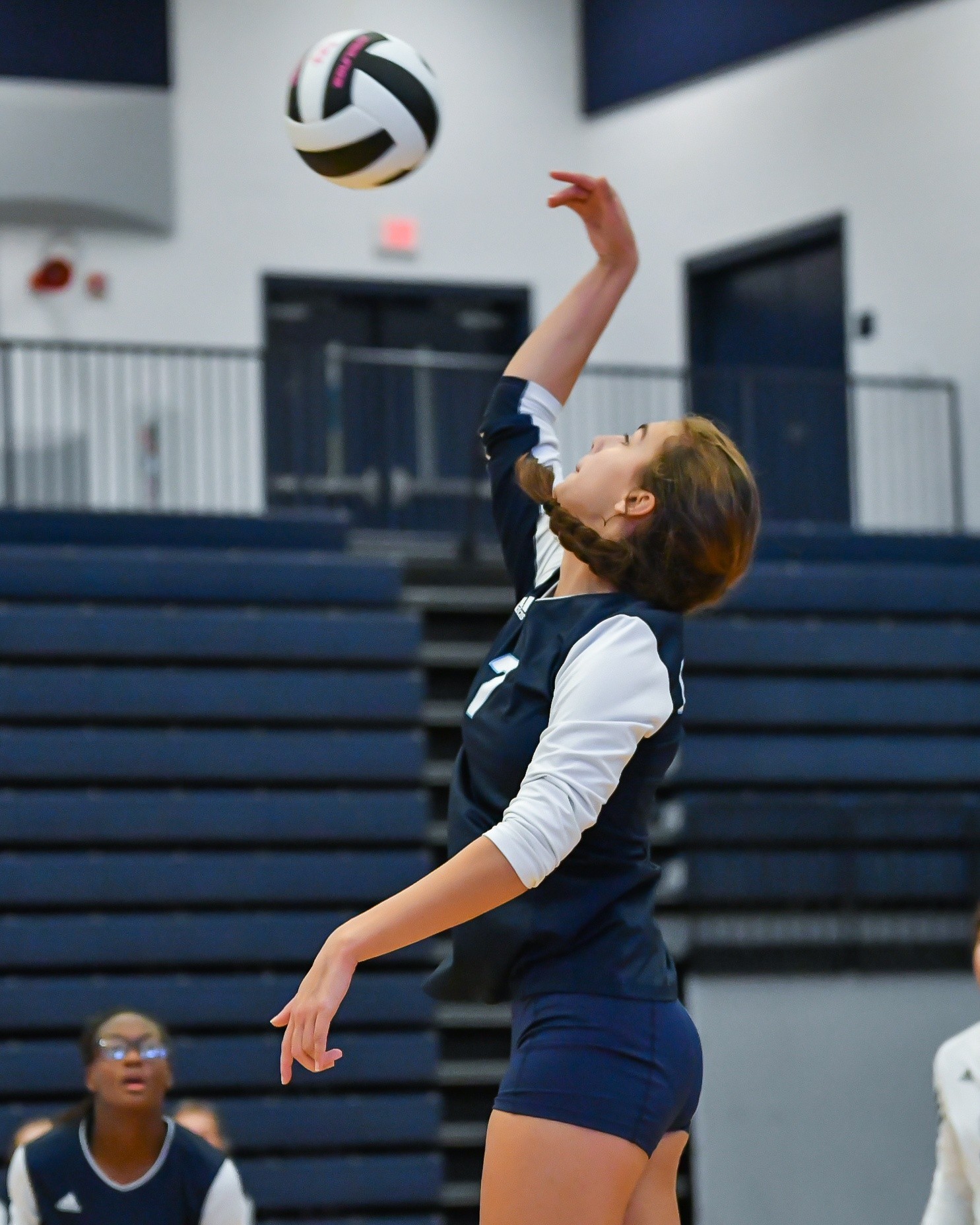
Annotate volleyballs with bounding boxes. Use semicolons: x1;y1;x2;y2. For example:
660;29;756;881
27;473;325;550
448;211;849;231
284;29;441;190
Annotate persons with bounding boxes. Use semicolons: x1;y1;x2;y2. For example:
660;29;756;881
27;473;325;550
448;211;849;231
0;1010;257;1225
914;912;980;1225
269;168;761;1225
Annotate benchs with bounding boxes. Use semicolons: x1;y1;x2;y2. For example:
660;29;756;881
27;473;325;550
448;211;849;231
0;505;454;1225
653;518;980;979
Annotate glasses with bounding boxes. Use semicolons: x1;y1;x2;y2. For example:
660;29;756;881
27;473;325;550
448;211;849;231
90;1044;169;1061
